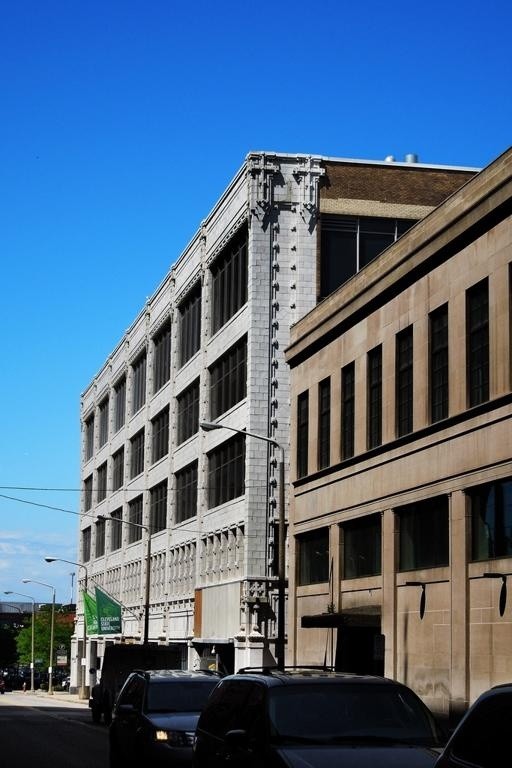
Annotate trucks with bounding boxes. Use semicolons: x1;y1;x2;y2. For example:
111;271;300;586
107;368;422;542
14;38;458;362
89;642;183;725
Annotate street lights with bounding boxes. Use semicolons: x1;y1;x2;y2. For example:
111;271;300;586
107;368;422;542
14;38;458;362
200;422;286;669
97;514;149;647
4;556;88;698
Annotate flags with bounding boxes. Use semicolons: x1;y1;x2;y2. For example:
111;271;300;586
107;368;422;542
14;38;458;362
81;588;98;636
94;585;124;635
